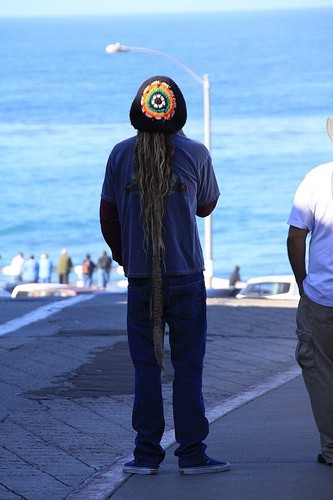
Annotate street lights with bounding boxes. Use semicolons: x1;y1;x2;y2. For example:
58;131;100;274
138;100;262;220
105;42;216;291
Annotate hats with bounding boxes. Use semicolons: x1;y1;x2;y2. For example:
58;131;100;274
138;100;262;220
130;76;188;133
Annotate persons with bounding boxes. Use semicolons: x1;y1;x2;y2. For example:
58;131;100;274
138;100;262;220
81;254;96;288
97;250;112;289
287;115;333;466
39;252;52;283
21;253;39;283
100;75;231;475
53;248;75;284
10;252;25;282
229;265;247;299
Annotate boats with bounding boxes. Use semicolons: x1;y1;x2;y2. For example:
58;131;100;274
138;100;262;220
0;267;310;301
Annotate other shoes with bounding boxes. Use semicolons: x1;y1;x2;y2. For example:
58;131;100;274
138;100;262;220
317;452;333;466
123;458;160;475
177;456;231;475
